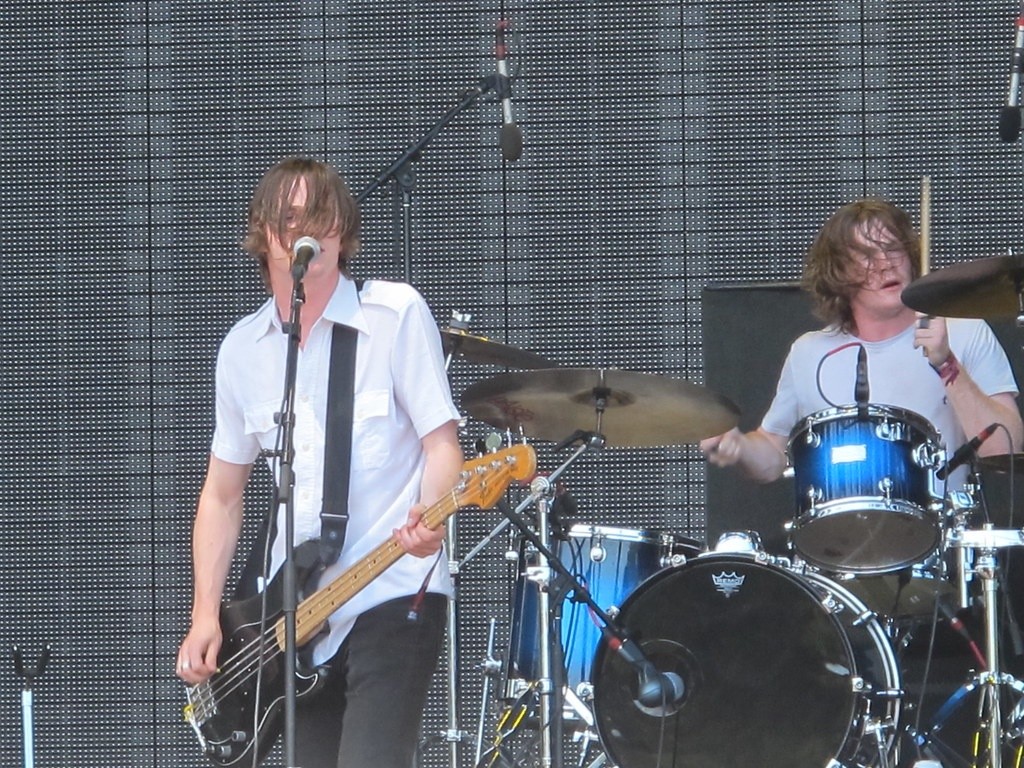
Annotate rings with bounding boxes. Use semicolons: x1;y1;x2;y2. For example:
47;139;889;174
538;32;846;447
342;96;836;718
920;318;930;329
181;661;191;671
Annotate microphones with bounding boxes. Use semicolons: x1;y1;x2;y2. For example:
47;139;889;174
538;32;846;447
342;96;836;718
292;236;321;277
854;346;870;418
496;21;523;161
936;423;1000;480
637;671;685;708
998;0;1024;143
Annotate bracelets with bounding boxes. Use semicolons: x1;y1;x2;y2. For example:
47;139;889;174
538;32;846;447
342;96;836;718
929;349;960;387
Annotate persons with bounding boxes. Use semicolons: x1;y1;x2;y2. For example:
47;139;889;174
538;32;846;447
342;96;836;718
175;156;464;768
700;197;1024;768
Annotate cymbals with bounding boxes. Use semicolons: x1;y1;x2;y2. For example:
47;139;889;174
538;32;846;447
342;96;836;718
459;365;744;451
899;252;1023;321
438;325;556;370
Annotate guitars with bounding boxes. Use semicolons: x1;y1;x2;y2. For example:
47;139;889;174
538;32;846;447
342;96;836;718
185;421;540;767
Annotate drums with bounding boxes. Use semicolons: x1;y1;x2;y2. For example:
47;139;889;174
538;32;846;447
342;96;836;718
787;403;946;576
498;520;709;727
591;551;902;768
